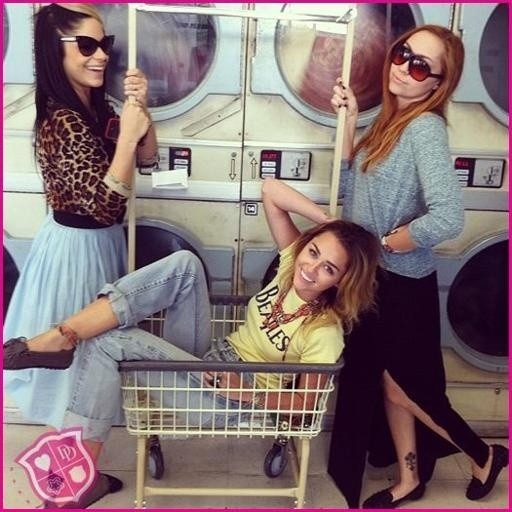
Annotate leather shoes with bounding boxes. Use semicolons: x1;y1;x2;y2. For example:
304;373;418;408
363;481;425;509
45;473;109;510
3;337;75;368
466;444;508;500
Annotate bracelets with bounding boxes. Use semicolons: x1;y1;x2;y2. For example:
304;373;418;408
248;392;265;409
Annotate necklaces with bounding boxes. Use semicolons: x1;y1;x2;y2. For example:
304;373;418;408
262;274;325;329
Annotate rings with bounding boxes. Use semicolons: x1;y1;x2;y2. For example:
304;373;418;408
209;376;222;389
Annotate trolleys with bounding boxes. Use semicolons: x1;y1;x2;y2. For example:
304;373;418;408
115;3;356;510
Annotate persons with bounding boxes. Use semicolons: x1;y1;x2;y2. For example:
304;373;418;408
2;2;158;491
2;180;380;510
328;25;510;510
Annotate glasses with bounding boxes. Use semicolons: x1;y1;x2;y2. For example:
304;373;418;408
60;35;114;56
390;44;441;81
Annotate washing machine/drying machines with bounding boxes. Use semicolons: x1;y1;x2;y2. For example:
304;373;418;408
112;202;236;362
241;206;344;399
4;4;90;191
429;209;507;439
455;3;508;209
5;195;100;432
100;5;239;199
243;1;450;203
